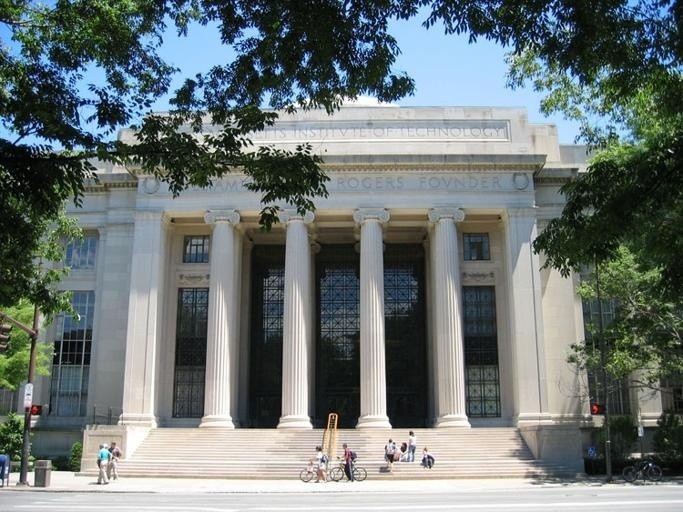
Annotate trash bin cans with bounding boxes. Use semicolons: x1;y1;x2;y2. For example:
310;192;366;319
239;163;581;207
34;460;53;487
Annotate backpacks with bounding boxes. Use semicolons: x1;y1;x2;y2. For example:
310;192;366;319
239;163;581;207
350;451;357;461
321;454;329;464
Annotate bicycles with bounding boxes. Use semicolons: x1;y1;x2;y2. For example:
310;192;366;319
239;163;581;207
299;459;335;483
620;454;664;483
329;456;367;481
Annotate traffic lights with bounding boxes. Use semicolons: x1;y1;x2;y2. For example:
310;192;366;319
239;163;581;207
30;404;42;416
589;401;604;415
0;320;12;352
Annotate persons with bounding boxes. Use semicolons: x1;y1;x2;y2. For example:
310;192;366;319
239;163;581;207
95;444;112;485
391;441;396;454
420;447;435;469
406;431;416;462
107;442;121;481
0;448;6;487
309;445;326;483
398;442;408;462
384;439;398;473
103;443;119;462
340;442;353;483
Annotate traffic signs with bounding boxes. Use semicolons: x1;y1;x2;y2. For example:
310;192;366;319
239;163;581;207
22;382;33;408
